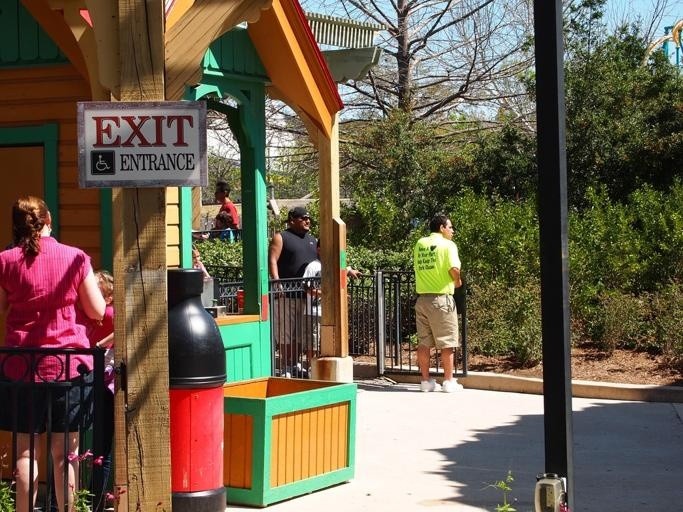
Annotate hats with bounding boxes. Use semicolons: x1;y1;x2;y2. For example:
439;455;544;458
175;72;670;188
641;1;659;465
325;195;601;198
288;207;309;217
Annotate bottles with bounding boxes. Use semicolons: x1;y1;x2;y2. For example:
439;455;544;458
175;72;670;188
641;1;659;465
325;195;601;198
167;267;229;512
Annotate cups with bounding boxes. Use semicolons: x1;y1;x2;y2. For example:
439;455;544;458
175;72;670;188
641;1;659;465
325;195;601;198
236;289;245;314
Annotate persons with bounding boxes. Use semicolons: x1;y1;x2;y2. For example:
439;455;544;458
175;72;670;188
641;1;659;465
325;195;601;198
413;215;464;393
0;195;114;512
214;182;240;244
192;248;211;278
269;207;318;378
303;238;362;378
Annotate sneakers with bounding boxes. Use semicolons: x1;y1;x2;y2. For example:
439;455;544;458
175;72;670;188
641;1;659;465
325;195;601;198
281;363;310;379
421;378;464;392
